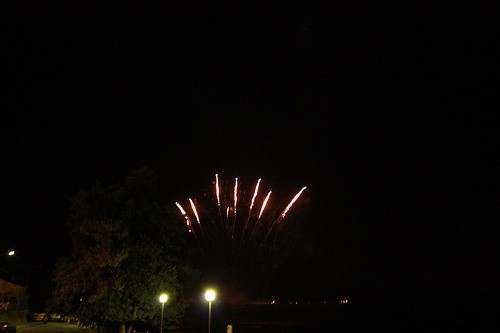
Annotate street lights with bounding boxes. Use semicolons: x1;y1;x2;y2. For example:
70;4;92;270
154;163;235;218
159;292;169;333
204;288;217;333
